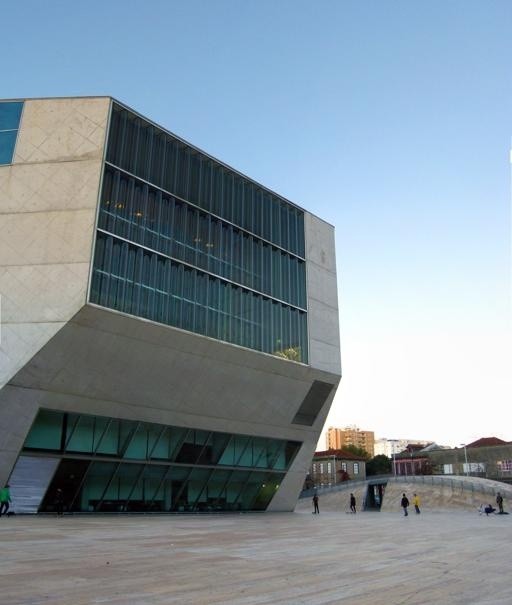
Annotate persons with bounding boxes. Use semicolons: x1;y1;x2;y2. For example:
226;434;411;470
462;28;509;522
496;491;504;513
0;484;12;516
412;493;421;514
312;492;320;514
401;493;410;517
349;492;357;513
56;488;65;520
